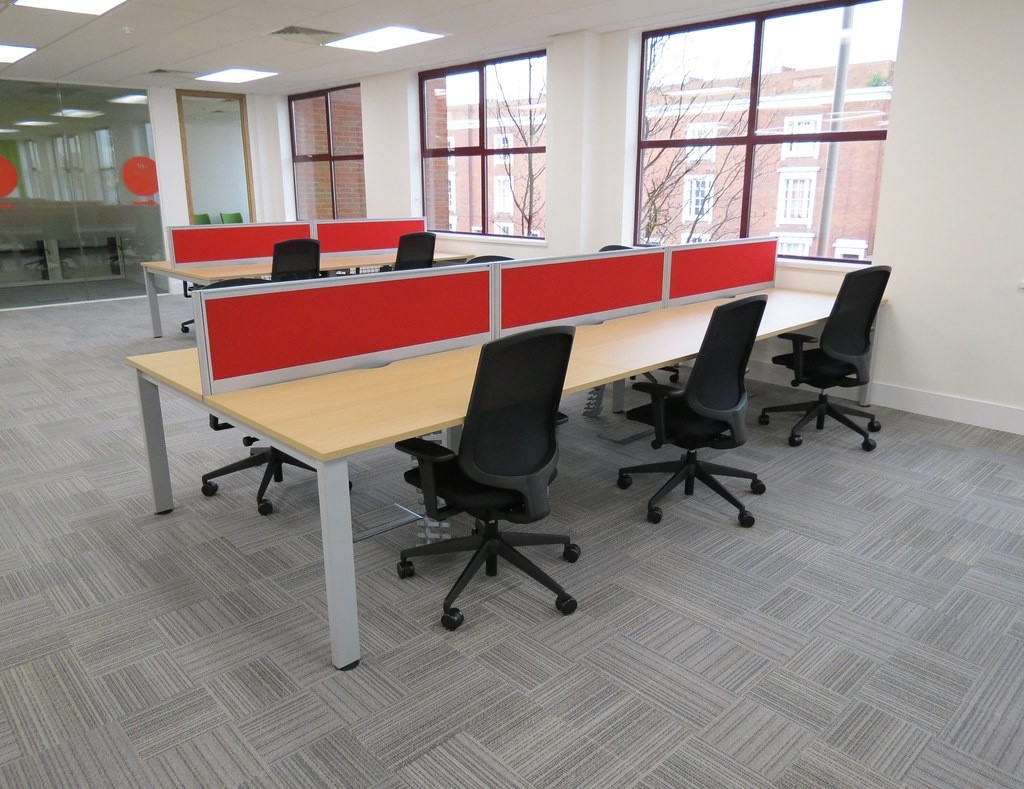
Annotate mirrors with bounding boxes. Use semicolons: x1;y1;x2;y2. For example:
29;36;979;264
175;89;257;225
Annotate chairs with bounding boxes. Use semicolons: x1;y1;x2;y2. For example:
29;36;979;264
202;278;352;515
759;265;892;452
182;279;206;333
395;326;580;632
267;239;328;282
379;233;436;272
220;213;243;224
599;245;681;383
194;214;211;225
617;294;767;528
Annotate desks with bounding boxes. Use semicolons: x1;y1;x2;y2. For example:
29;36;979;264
141;217;475;338
124;234;839;670
